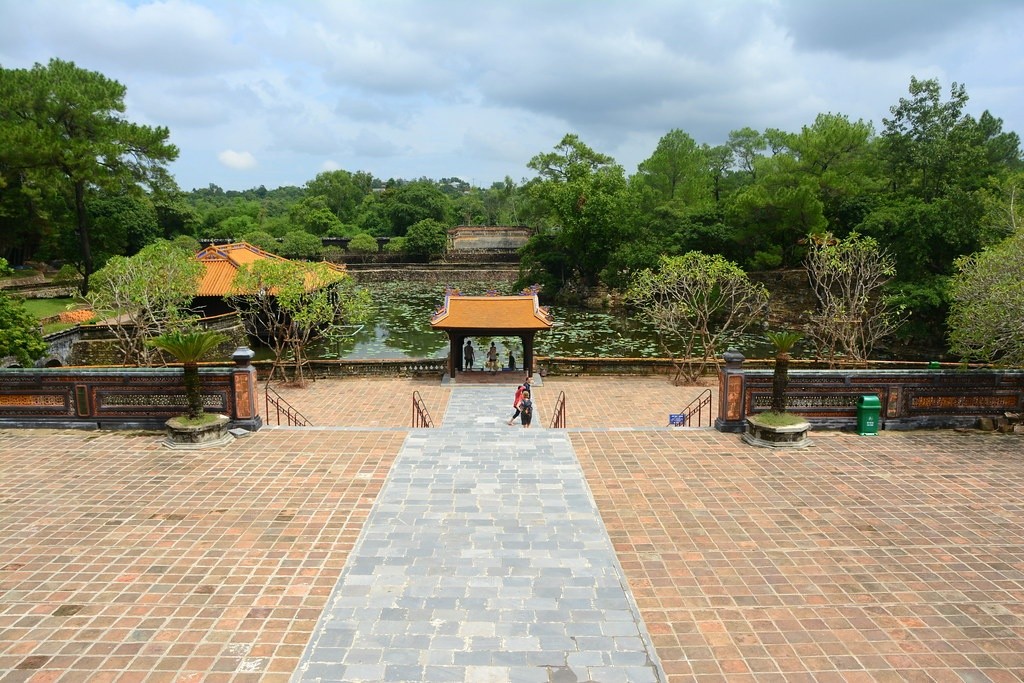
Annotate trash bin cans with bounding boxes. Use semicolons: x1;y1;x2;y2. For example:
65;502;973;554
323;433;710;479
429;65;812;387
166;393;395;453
857;396;881;436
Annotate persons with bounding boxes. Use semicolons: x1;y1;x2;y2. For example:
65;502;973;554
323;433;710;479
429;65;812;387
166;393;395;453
509;377;533;428
464;341;476;371
487;341;497;371
508;351;515;368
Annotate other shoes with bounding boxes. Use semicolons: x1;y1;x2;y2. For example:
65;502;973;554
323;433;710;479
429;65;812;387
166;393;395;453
508;422;513;426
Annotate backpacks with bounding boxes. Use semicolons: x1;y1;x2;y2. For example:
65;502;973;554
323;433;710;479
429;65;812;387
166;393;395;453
522;399;531;414
